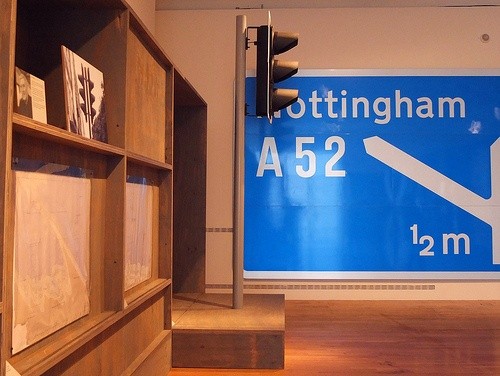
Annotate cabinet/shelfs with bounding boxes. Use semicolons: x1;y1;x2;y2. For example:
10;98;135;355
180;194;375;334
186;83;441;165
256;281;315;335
0;0;173;376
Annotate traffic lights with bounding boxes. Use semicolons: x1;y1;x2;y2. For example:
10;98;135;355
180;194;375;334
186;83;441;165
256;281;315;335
78;64;97;124
255;11;301;119
